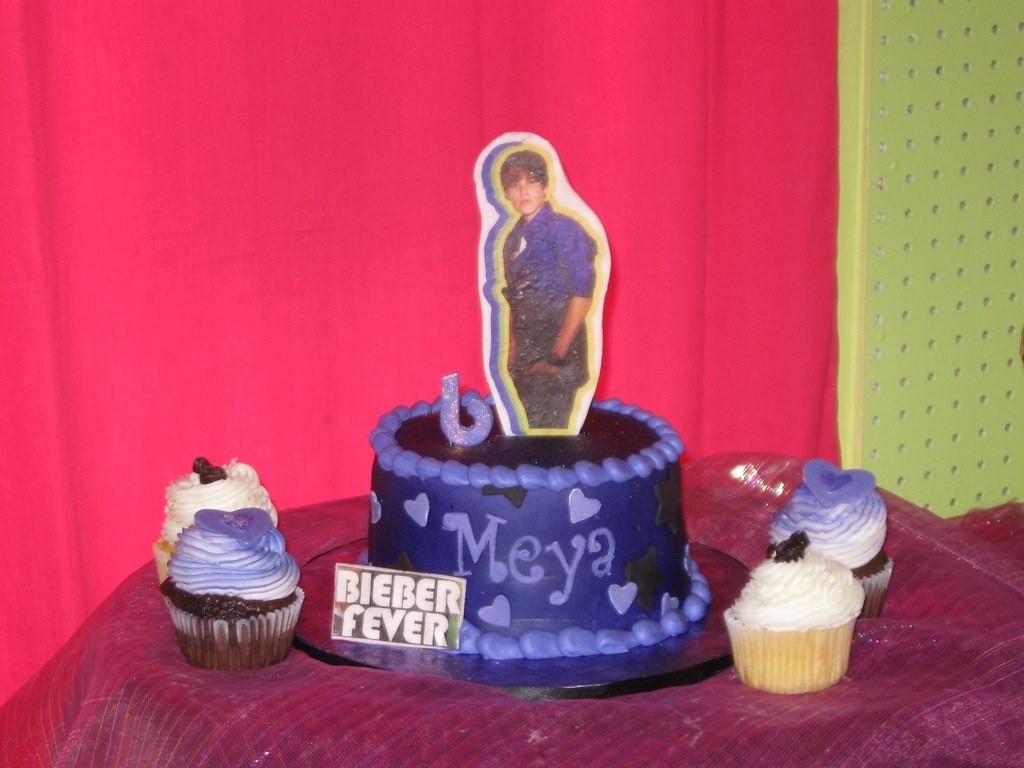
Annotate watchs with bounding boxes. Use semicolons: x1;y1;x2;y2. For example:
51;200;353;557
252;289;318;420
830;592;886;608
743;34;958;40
544;353;562;366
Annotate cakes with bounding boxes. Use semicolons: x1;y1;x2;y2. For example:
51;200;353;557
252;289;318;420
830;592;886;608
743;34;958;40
357;131;713;661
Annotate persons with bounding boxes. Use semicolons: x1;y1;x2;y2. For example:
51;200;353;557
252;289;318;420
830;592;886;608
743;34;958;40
500;149;598;431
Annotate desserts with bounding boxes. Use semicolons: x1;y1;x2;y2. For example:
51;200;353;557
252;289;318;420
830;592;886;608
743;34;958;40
769;459;893;620
154;457;307;672
721;531;865;695
